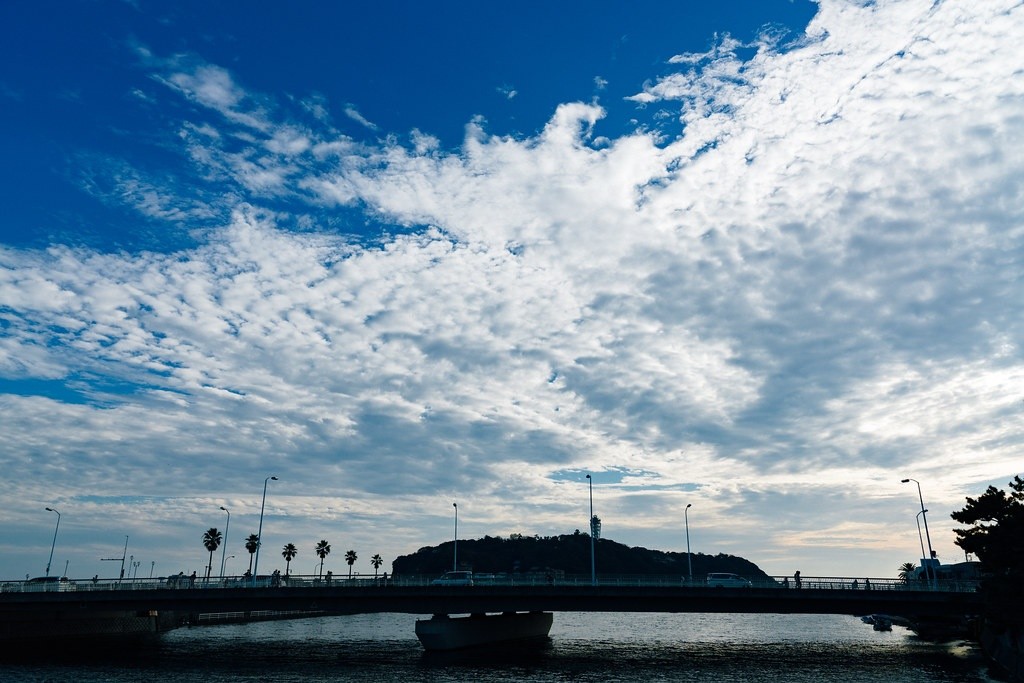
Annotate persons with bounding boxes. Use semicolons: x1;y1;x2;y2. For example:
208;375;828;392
866;578;870;590
188;571;197;589
326;571;332;585
795;572;802;589
706;574;713;585
176;571;183;588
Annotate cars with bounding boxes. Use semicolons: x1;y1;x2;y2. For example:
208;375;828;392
0;582;23;593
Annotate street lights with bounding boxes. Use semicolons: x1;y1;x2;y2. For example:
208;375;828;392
685;504;696;582
915;508;931;590
453;503;457;571
45;507;61;576
901;479;937;588
253;476;278;587
220;506;231;575
119;535;129;582
222;555;235;580
314;563;324;580
586;474;595;582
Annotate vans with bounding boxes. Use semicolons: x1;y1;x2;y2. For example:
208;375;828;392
706;572;752;589
439;571;473;586
23;575;70;592
